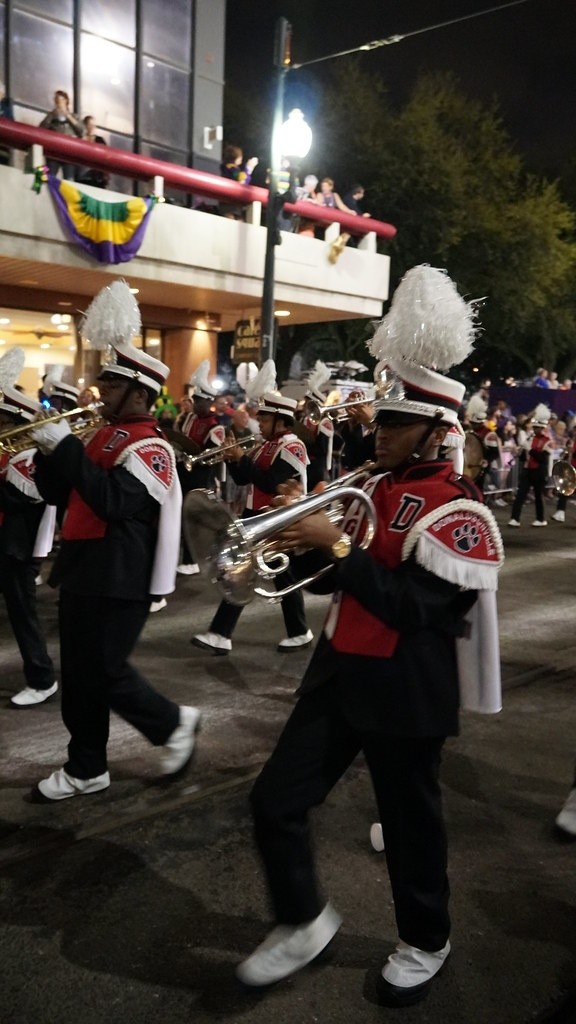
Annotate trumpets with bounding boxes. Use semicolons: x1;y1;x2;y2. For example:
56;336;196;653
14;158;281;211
305;398;376;426
552;461;576;496
0;401;106;456
182;460;377;607
183;431;263;472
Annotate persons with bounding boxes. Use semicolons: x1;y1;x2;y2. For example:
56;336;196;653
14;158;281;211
36;341;203;802
266;157;372;248
74;116;110;189
219;144;258;222
39;91;87;183
17;368;576;613
0;386;58;708
240;354;503;1002
0;84;15;165
195;358;314;653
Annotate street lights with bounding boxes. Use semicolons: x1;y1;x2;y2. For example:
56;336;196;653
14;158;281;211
257;107;313;377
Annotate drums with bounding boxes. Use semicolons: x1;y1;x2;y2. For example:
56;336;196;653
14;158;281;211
160;426;196;478
463;430;486;483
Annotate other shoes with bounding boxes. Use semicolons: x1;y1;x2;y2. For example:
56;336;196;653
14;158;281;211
159;706;202;777
176;564;200;575
377;937;449;1005
149;598;168;612
278;629;315;651
35;575;43;585
555;788;576;836
235;906;343;986
486;489;568;527
37;767;110;800
189;631;232;655
10;681;59;708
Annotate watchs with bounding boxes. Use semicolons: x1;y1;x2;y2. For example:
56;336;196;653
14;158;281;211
331;533;353;559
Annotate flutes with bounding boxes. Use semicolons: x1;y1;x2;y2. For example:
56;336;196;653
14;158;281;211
508;432;535;465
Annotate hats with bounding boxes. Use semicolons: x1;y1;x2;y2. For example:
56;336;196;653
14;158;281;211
190;360;216;400
531;403;551;429
78;281;170;396
43;363;80;403
468;394;486;423
369;262;477;426
236;359;331;421
0;349;42;422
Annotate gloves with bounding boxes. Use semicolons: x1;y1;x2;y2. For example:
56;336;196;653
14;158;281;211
26;410;74;456
